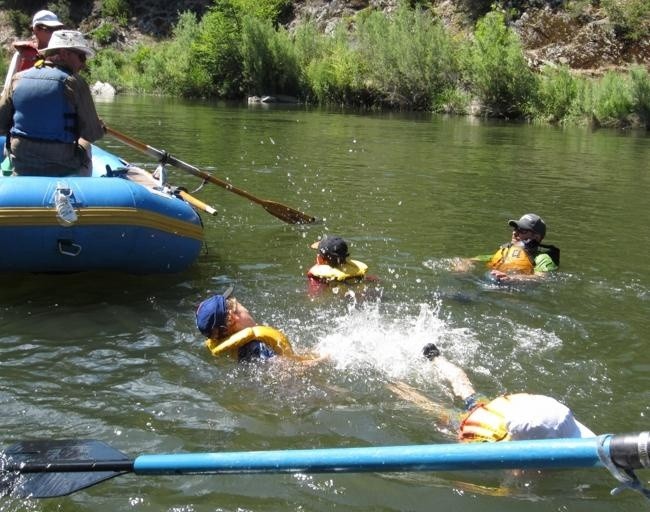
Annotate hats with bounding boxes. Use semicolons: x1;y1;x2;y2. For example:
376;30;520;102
311;236;350;260
196;287;234;340
507;396;597;439
37;29;97;59
33;10;64;27
509;213;546;237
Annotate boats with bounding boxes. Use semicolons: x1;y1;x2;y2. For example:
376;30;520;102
0;133;203;274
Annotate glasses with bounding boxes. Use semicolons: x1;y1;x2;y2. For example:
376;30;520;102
39;25;58;30
66;47;86;62
512;224;529;234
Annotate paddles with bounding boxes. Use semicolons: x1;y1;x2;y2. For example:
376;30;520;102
0;434;648;501
105;125;317;223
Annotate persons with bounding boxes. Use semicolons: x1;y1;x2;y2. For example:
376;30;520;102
194;283;334;378
439;213;561;305
387;340;600;479
305;234;383;310
1;8;65;109
7;28;108;175
